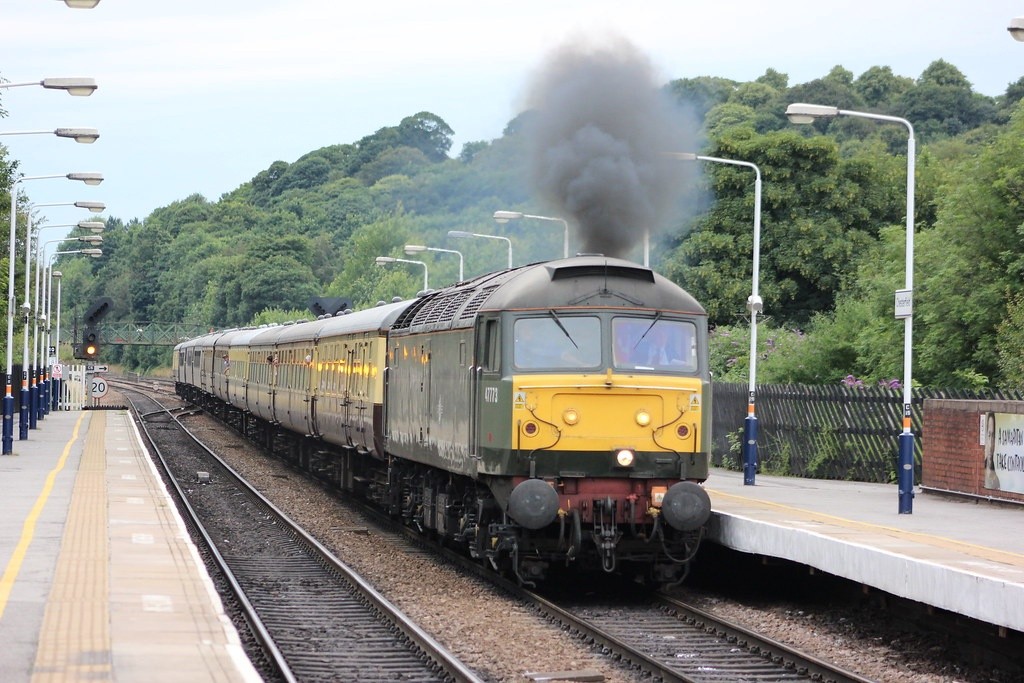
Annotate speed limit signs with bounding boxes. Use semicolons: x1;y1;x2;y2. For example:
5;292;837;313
90;376;109;398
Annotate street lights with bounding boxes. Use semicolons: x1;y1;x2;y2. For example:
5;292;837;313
51;271;63;412
661;152;764;485
493;210;569;260
785;103;915;515
404;245;464;282
30;221;106;425
376;256;428;291
3;171;105;455
39;235;103;420
44;248;103;415
448;230;513;267
18;200;106;441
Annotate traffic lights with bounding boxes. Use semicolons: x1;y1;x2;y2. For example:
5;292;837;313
82;328;99;360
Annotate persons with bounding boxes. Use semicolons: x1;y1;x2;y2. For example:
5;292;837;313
632;326;680;369
222;355;230;377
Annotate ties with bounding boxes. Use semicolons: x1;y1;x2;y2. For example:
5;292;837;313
652;350;659;367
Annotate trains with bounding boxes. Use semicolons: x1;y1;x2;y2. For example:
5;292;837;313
172;248;713;594
